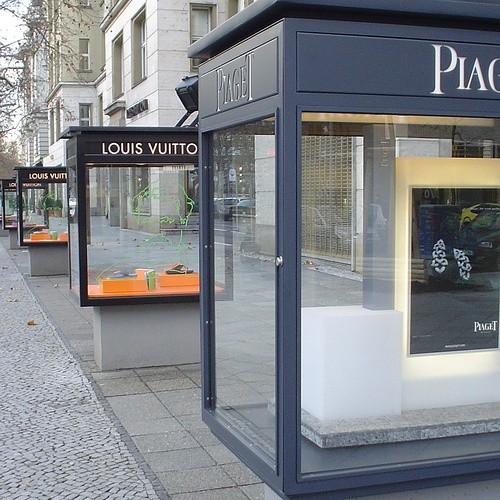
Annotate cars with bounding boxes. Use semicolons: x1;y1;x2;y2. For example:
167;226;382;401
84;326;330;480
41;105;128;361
455;208;500;271
460;203;500;224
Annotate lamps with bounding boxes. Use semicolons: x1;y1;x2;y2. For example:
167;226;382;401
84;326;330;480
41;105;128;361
172;74;198;129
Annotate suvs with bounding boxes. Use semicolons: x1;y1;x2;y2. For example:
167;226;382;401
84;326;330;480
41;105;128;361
214;197;256;222
